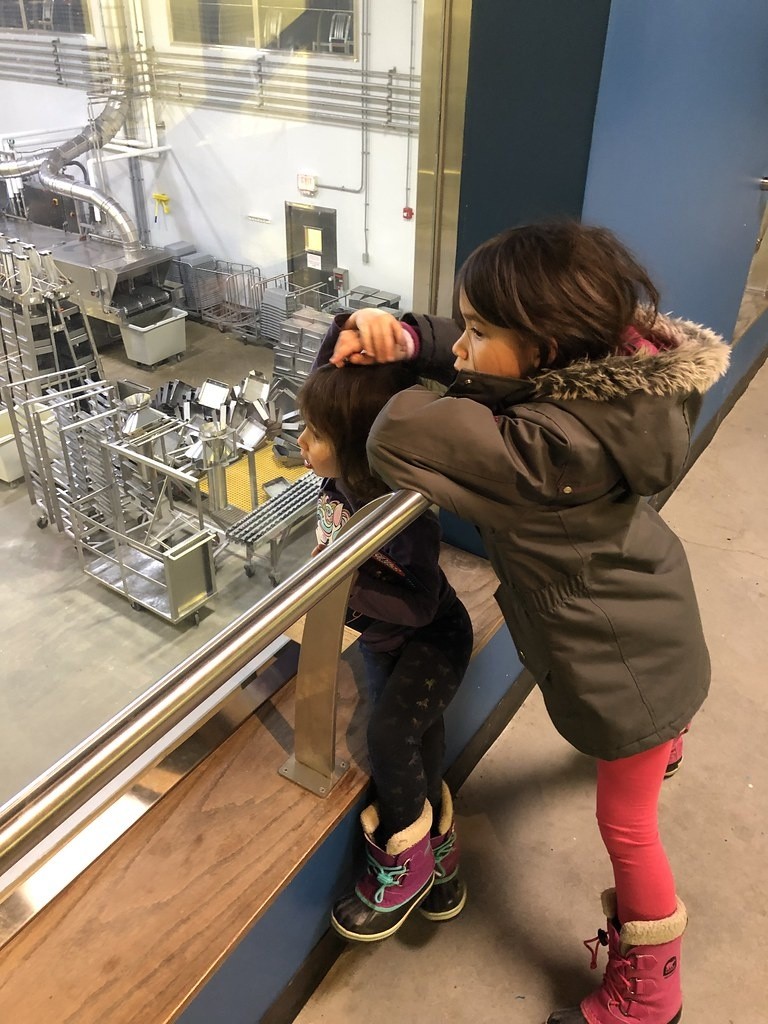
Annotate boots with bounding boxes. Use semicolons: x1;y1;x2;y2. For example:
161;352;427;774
662;734;683;776
547;888;689;1024
330;798;439;941
418;781;466;920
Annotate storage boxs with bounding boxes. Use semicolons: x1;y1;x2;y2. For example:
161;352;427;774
0;403;60;488
120;306;189;370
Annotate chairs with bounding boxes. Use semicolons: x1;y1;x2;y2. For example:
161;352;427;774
31;0;55;30
313;13;351;52
247;11;281;49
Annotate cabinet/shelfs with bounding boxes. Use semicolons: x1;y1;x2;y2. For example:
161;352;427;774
68;484;217;628
0;287;107;405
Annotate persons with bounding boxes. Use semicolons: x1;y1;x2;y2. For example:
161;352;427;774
331;221;736;1024
295;308;475;944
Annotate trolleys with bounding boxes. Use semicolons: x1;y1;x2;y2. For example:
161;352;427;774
6;371;158;550
191;260;260;333
67;441;215;631
